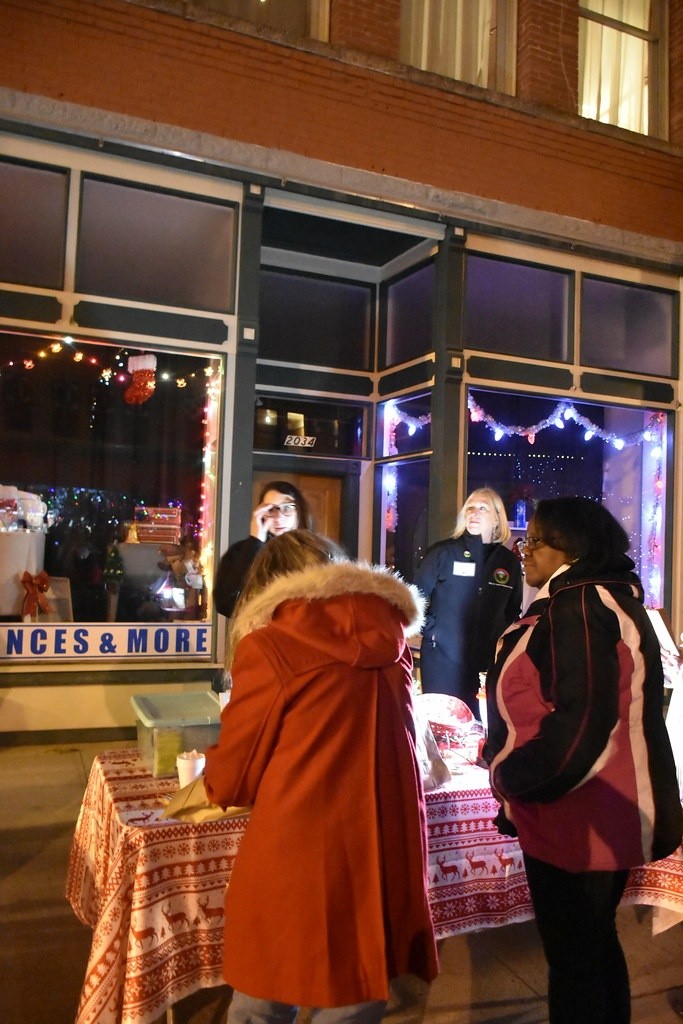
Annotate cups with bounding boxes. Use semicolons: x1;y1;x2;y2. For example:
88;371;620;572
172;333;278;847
177;752;206;791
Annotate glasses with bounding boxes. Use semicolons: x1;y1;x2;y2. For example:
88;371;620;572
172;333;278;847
263;502;298;518
515;535;547;552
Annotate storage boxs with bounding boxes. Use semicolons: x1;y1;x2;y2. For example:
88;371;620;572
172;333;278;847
131;690;224;779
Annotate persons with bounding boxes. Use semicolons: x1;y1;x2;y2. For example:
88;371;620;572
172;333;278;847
204;527;441;1024
479;497;682;1024
214;481;315;618
416;486;524;721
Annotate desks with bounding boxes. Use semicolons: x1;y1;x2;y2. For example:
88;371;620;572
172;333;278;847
94;749;683;1024
